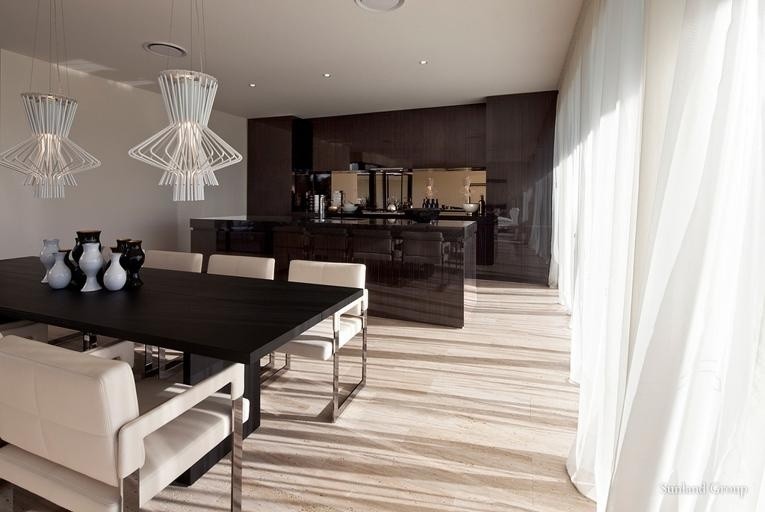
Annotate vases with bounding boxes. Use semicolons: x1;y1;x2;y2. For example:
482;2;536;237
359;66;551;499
39;230;145;292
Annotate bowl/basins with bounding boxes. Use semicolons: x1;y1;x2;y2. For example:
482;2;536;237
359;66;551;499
404;207;441;223
463;203;479;213
342;206;358;211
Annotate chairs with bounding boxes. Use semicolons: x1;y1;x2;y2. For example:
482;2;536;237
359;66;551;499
1;335;251;510
1;320;104;355
258;259;370;422
158;254;277;379
83;251;203;375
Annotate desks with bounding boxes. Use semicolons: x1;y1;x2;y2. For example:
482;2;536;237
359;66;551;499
1;257;364;487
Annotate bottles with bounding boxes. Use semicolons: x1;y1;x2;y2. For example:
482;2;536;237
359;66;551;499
422;199;451;210
333;191;341;207
478;195;485;215
40;230;146;292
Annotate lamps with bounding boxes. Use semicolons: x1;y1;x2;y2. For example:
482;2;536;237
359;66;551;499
1;1;103;199
127;0;243;200
